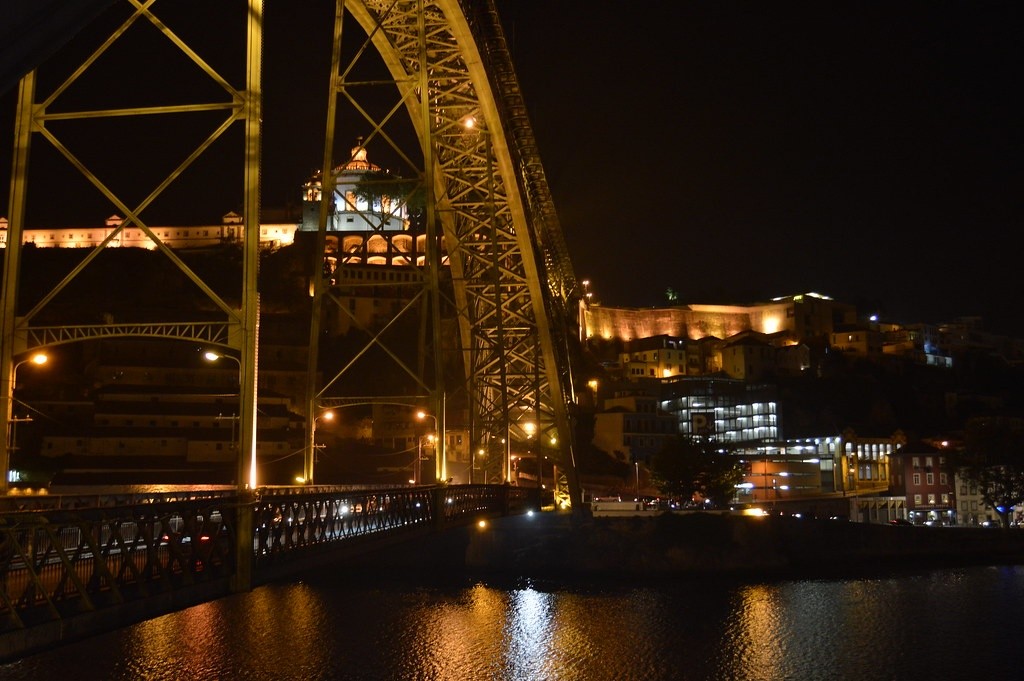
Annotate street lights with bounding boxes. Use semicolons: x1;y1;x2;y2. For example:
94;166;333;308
5;352;49;493
204;351;254;488
311;411;334;485
416;410;437;481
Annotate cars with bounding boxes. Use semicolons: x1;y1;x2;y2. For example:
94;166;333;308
889;517;913;526
979;520;995;527
633;496;662;511
165;515;231;566
922;518;943;526
